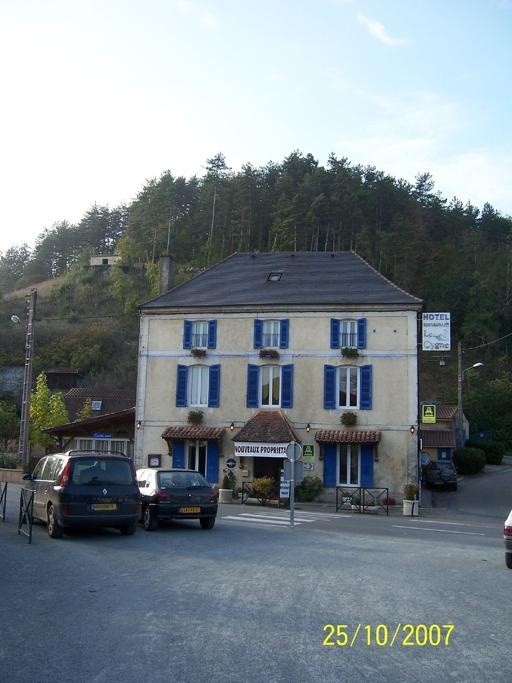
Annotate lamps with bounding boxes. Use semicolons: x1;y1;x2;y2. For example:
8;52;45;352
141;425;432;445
230;422;234;430
410;425;415;434
306;424;310;432
137;421;141;429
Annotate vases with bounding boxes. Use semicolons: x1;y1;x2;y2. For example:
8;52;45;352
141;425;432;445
363;502;378;513
384;505;393;510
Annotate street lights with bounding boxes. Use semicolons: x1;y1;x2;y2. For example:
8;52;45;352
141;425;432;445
457;363;485;448
11;315;35;463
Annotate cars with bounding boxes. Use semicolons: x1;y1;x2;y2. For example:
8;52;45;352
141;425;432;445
136;468;218;531
504;508;512;568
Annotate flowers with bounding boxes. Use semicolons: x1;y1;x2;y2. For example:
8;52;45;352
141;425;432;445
383;497;396;505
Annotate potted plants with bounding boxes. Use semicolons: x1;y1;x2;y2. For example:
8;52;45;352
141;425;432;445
340;347;359;358
219;475;235;503
340;412;358;426
253;477;275;504
259;349;278;359
403;485;418;516
342;491;352;504
190;349;205;358
187;411;203;426
351;494;361;512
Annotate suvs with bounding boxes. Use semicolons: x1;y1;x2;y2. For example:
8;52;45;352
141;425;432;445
423;460;457;491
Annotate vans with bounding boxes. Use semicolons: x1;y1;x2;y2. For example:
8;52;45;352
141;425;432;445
19;450;142;538
